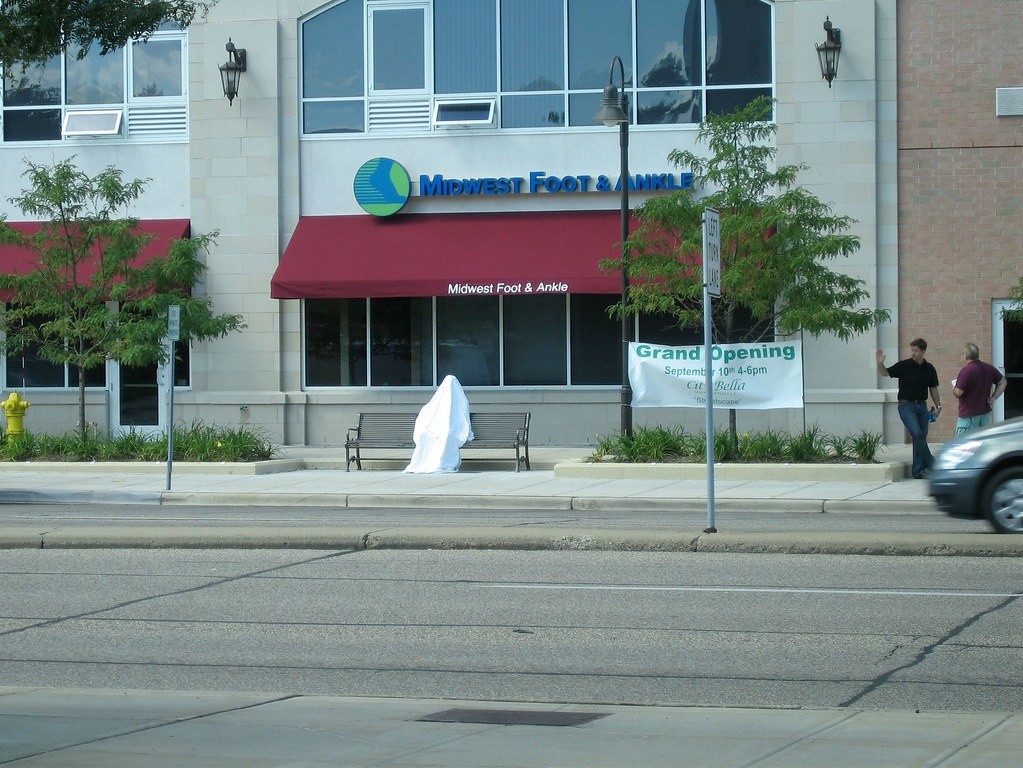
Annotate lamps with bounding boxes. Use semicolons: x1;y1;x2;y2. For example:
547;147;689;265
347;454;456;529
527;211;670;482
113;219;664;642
813;15;842;88
217;36;247;107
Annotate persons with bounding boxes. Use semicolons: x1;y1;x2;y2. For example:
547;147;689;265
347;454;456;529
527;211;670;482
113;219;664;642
876;338;942;479
952;342;1007;436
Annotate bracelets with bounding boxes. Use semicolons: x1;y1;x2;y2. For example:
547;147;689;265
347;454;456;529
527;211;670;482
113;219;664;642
936;406;942;409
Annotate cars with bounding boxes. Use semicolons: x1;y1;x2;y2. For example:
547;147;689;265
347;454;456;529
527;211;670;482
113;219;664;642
925;417;1023;534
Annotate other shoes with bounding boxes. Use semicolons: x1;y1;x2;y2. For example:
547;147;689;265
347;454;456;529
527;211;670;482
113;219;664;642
915;471;929;479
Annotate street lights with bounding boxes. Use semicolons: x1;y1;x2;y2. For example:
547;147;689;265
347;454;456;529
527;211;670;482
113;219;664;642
591;55;634;437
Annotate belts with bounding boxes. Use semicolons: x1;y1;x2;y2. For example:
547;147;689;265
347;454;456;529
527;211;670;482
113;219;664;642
907;398;925;403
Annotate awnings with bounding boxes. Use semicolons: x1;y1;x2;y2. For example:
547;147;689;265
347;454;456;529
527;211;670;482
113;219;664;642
0;218;191;302
270;207;776;301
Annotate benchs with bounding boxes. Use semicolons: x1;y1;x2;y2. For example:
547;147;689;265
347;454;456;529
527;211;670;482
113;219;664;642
344;412;531;473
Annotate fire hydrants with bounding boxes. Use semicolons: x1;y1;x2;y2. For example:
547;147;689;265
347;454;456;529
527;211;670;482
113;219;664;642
2;395;29;446
